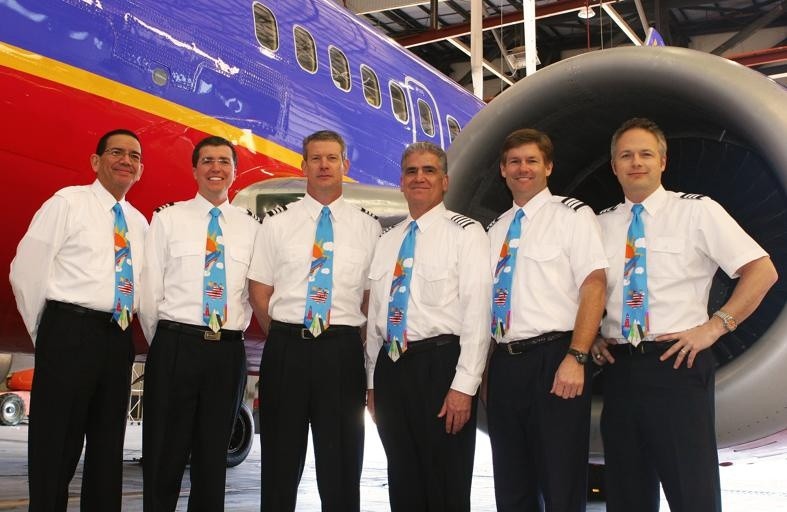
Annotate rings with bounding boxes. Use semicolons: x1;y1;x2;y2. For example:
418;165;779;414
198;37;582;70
594;352;602;360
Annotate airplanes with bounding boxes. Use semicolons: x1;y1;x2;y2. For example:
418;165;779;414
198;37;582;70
0;0;787;493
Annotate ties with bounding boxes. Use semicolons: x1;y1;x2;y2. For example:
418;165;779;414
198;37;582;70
492;209;523;342
202;207;228;335
385;222;418;362
303;207;334;340
621;205;650;346
112;204;135;331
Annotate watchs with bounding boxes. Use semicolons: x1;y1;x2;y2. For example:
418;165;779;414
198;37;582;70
714;311;738;333
567;348;588;365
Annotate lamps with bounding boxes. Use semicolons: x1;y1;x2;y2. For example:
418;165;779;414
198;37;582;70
505;23;542;70
578;7;595;19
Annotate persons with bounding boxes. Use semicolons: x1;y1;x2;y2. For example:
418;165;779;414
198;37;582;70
245;130;383;512
137;135;264;512
9;129;150;512
588;118;778;512
475;130;612;512
367;143;493;512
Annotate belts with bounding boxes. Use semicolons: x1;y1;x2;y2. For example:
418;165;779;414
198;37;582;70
493;331;572;354
270;324;361;339
380;338;456;355
158;318;245;341
46;301;131;325
606;342;672;357
